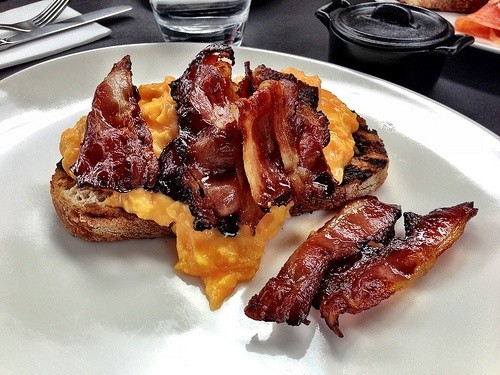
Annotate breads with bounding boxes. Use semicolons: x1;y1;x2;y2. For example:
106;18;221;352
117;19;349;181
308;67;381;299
396;0;490;14
51;110;390;242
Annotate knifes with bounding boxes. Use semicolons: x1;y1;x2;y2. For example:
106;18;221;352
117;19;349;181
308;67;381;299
0;5;133;51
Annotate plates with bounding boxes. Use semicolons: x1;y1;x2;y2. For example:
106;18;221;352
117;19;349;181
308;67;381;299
375;0;500;53
0;0;113;69
0;42;500;375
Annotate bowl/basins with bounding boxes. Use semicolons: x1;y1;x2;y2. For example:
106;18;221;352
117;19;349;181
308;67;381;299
315;0;475;95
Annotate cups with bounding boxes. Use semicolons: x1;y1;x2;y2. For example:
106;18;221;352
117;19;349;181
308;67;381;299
149;0;251;46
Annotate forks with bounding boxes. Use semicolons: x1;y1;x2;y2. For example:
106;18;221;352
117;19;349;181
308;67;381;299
0;0;69;33
